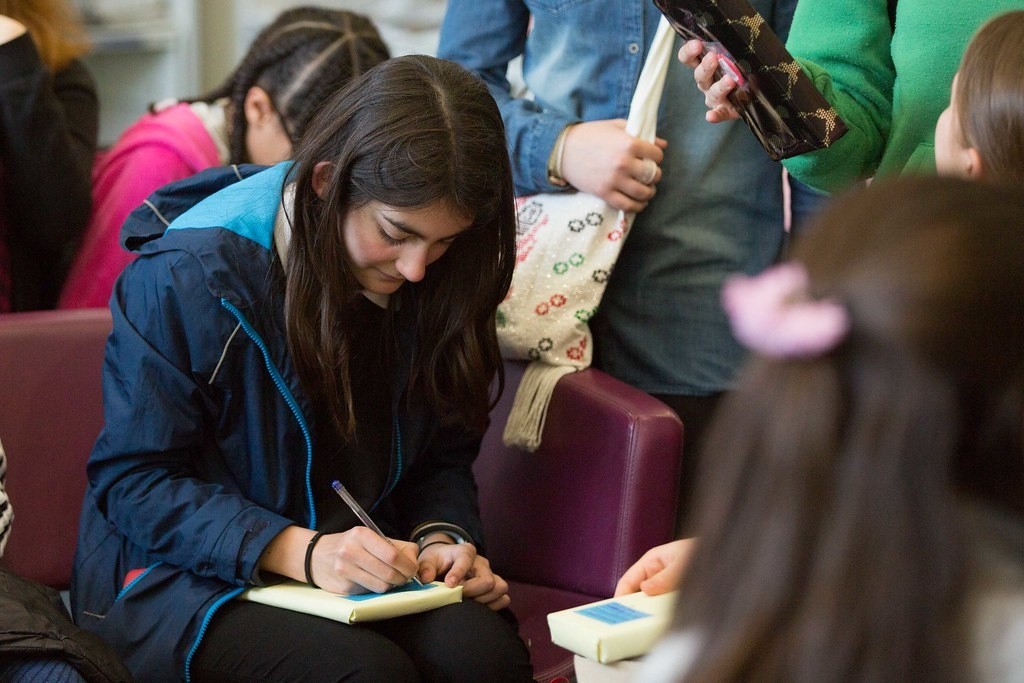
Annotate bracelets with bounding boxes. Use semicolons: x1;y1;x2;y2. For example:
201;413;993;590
417;542;451;558
305;529;335;587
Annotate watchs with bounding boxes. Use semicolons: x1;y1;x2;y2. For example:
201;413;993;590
548;124;570;187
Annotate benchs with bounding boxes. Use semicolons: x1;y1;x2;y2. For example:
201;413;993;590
1;298;684;683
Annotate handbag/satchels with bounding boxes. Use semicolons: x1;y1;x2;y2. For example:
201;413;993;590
654;0;849;162
496;191;640;451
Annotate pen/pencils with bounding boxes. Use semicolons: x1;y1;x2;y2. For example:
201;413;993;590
331;479;424;588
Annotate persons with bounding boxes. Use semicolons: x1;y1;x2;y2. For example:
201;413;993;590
58;7;393;315
434;0;798;541
933;11;1024;192
71;55;537;683
675;0;1024;197
0;428;86;682
0;0;101;315
612;174;1024;683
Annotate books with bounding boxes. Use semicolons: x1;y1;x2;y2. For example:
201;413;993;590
653;0;849;163
